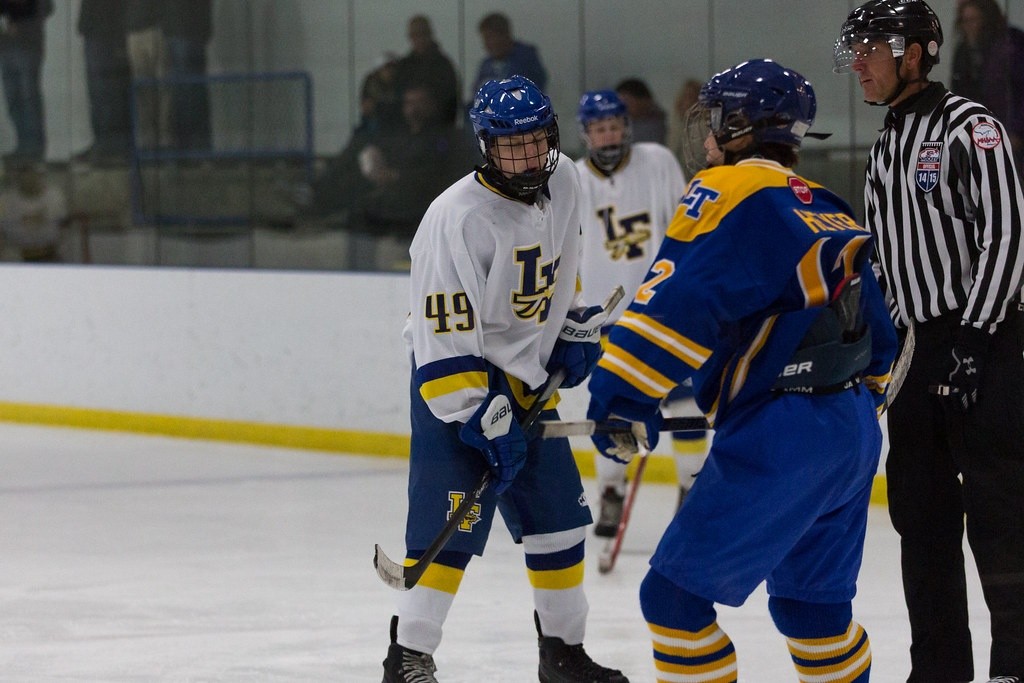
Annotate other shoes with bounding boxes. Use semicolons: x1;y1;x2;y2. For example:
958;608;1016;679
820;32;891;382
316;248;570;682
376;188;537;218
986;676;1019;683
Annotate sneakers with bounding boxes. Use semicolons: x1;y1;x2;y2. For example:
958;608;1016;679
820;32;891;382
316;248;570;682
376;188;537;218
384;617;440;683
595;486;623;536
533;611;630;683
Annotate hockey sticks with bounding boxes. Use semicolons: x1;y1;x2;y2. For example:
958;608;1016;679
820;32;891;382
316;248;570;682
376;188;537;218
533;317;918;443
370;284;626;593
596;448;650;574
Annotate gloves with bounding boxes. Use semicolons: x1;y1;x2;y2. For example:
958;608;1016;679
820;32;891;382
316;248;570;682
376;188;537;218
459;389;526;496
585;395;661;464
932;325;992;413
545;305;604;389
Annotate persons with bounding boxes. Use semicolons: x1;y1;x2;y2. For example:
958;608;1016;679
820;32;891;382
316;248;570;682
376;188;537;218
614;79;666;144
832;0;1024;683
380;74;632;683
0;0;55;160
311;15;459;238
586;58;899;683
471;13;549;102
70;0;213;166
950;0;1024;188
570;90;708;538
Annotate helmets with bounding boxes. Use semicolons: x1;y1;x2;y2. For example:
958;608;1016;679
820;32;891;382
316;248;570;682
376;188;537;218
684;59;817;177
841;0;943;70
468;74;560;202
577;88;628;165
